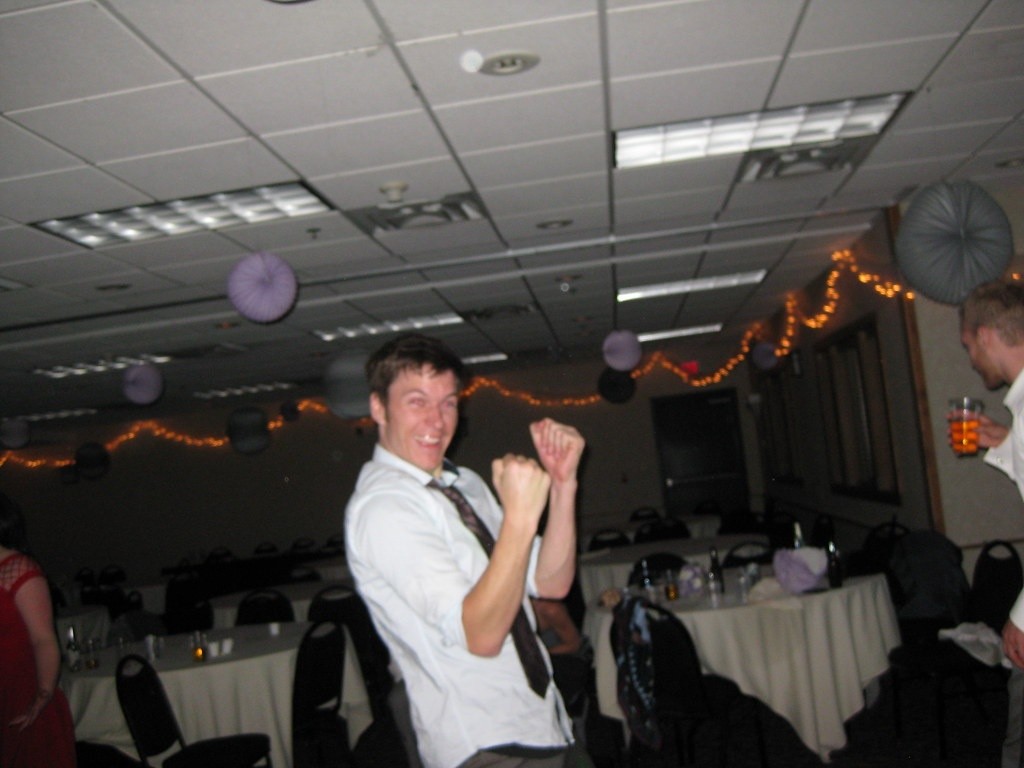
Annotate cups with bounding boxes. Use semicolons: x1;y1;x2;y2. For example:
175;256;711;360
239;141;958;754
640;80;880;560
222;637;234;654
207;641;219;658
947;396;983;454
269;623;280;636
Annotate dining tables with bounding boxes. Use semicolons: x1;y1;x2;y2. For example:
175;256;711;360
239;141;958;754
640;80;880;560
56;509;906;768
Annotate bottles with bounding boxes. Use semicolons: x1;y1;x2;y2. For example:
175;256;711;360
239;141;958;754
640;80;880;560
710;547;724;591
640;559;652;589
665;569;677;600
794;522;804;548
828;540;843;589
67;624;82;672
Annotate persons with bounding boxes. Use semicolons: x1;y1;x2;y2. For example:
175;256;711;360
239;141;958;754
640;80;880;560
0;546;78;768
946;278;1024;768
343;330;585;768
